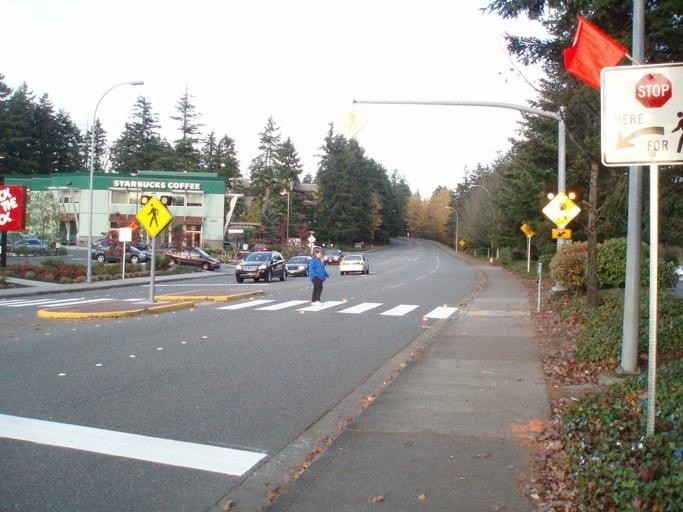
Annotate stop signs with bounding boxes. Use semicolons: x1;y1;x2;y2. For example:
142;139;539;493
631;72;674;111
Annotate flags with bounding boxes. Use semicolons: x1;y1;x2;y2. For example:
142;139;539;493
563;16;628;90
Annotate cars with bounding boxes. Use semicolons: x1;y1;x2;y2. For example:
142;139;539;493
0;231;78;258
223;241;369;284
91;235;223;271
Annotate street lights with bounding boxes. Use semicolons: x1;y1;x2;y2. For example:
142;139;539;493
86;79;144;283
278;190;290;245
467;183;501;264
444;206;459;256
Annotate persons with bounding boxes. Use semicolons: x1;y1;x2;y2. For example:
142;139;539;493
308;248;330;306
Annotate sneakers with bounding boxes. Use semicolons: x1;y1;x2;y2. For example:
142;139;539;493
311;301;322;307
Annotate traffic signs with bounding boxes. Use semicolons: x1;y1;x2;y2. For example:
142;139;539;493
595;61;683;169
